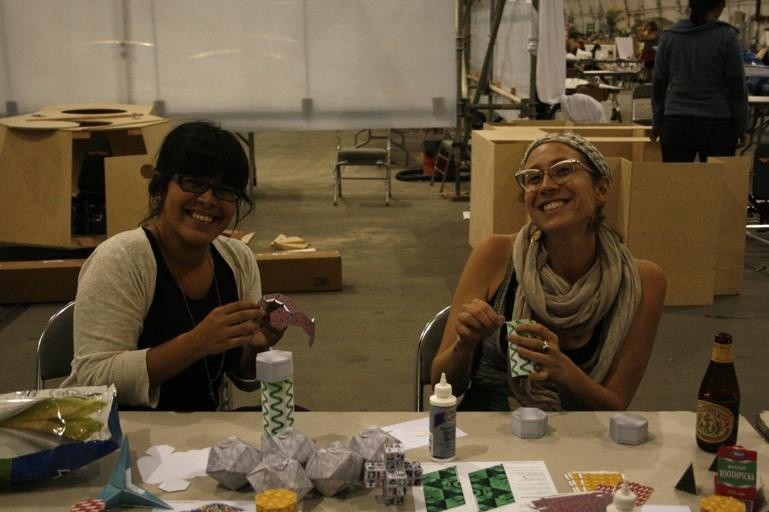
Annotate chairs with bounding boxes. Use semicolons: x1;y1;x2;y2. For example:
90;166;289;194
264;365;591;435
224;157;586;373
332;124;412;206
36;299;73;390
418;303;474;412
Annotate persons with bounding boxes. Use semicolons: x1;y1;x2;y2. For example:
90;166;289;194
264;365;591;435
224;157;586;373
650;1;749;163
565;27;601;56
428;133;666;411
640;22;660;83
55;121;309;412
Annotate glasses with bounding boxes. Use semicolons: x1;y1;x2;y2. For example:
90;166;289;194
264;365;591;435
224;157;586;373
516;158;597;192
170;174;241;202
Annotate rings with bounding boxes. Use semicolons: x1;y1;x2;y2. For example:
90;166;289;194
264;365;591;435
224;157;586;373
542;339;550;354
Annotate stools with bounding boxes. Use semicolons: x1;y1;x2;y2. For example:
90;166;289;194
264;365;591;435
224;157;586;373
599;83;627;120
739;94;769;155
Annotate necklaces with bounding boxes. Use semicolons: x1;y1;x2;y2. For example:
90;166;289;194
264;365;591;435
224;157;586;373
152;222;226;401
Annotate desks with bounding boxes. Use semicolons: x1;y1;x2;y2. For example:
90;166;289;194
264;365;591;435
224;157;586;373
2;409;768;511
585;69;636;87
744;65;769;89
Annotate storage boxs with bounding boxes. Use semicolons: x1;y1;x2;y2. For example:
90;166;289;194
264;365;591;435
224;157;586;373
254;248;343;296
1;258;84;300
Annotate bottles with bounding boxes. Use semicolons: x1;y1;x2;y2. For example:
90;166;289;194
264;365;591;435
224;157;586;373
695;332;741;453
428;372;457;463
606;479;641;512
